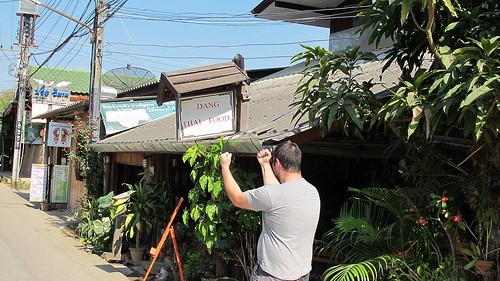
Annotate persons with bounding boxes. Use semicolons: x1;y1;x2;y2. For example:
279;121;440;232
220;139;321;281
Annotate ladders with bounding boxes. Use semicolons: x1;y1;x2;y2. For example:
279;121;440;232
142;197;188;281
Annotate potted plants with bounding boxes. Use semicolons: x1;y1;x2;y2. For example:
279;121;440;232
110;169;169;263
459;207;498;277
